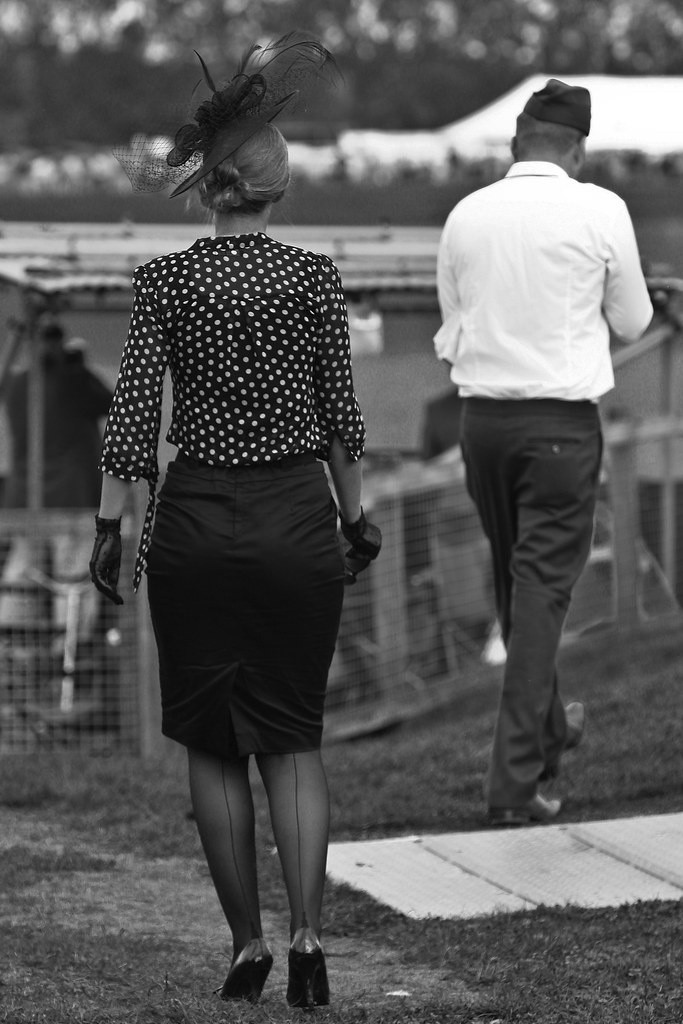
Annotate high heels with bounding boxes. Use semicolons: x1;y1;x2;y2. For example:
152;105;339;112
287;929;330;1011
213;937;273;1003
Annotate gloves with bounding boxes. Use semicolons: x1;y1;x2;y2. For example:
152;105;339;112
338;505;382;565
88;513;124;607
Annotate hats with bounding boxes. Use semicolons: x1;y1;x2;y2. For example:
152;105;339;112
523;78;592;136
109;28;346;199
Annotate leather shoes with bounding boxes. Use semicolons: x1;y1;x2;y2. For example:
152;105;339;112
536;702;584;782
489;788;561;826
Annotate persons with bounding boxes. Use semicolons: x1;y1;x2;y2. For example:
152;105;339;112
1;328;113;509
434;79;655;825
89;32;383;1012
